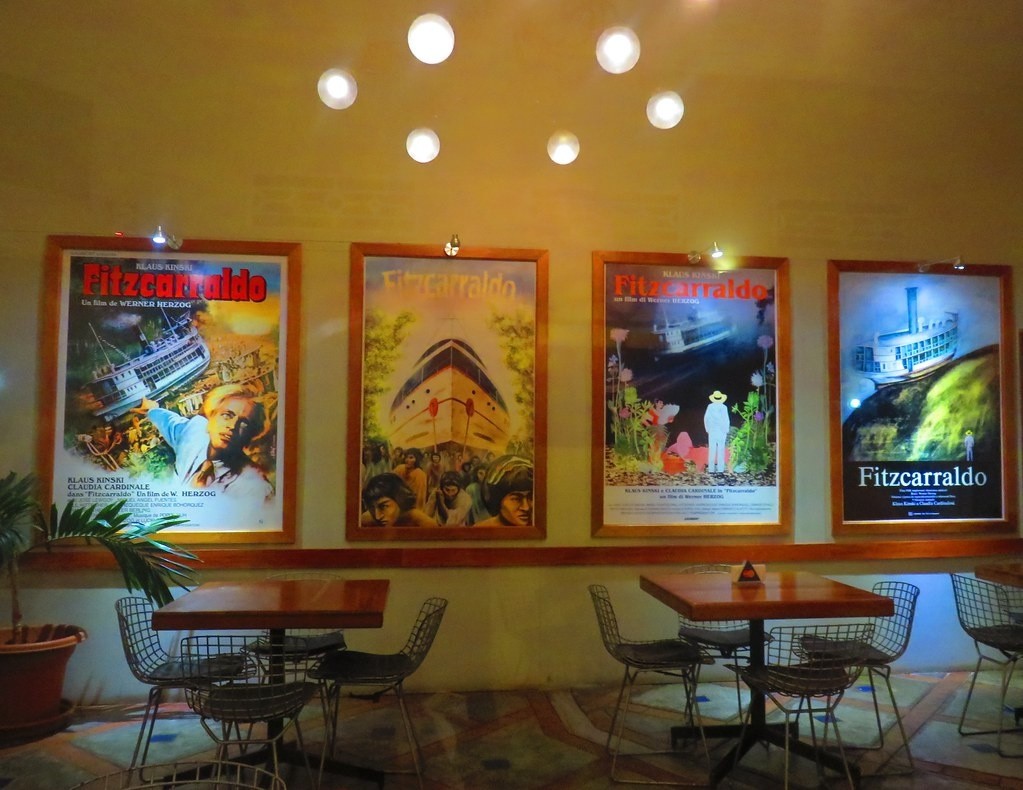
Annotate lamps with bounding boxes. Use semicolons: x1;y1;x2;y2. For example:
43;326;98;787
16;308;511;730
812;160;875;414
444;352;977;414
918;255;964;273
445;233;461;257
687;241;724;265
153;225;183;250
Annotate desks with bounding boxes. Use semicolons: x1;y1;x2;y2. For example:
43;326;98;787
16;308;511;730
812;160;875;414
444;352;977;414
151;579;390;790
640;569;894;790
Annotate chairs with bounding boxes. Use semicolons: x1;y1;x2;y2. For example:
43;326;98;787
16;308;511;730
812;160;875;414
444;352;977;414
68;571;449;790
723;623;876;790
792;581;920;780
588;584;716;788
950;573;1023;758
677;565;775;747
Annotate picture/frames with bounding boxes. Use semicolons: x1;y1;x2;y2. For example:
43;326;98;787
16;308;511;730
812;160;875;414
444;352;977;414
591;251;795;538
33;235;301;544
346;242;549;542
826;259;1019;537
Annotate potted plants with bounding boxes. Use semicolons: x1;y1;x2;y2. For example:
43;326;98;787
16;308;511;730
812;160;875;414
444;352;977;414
0;470;203;744
